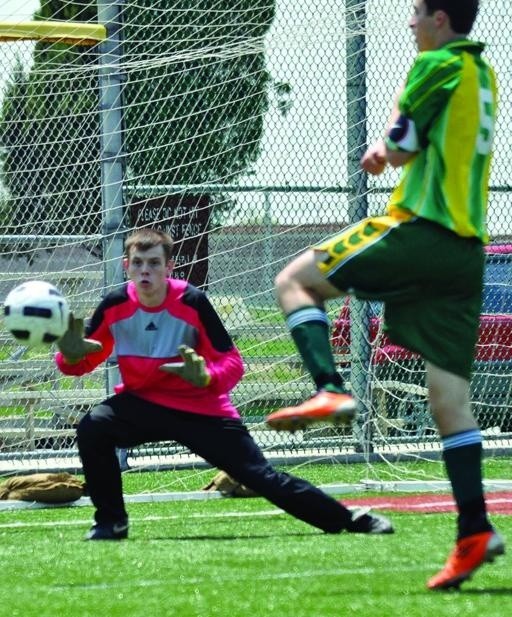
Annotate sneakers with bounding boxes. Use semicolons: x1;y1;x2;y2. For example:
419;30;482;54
268;388;358;433
428;533;504;591
345;512;393;534
83;522;127;540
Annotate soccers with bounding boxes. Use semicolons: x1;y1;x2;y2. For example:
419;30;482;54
1;280;70;346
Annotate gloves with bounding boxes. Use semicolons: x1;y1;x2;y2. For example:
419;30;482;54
160;345;211;387
58;313;102;365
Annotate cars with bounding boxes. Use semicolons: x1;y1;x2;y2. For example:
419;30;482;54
333;242;510;436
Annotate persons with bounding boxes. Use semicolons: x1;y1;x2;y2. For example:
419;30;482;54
56;229;398;539
261;0;508;591
